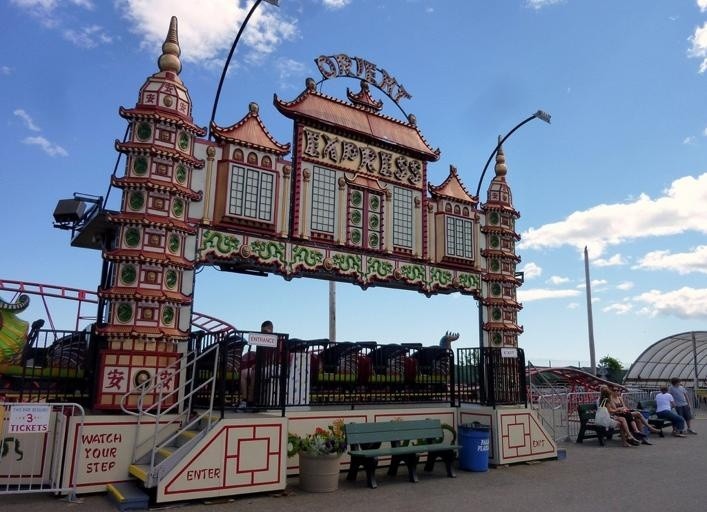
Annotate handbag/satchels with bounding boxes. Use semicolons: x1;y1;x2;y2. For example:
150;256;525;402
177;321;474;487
594;406;612;428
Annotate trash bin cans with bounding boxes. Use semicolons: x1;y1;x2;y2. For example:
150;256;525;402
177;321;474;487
457;423;491;472
637;410;649;433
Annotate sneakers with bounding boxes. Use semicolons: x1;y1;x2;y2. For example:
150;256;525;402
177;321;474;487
671;427;699;438
239;401;248;408
621;425;663;447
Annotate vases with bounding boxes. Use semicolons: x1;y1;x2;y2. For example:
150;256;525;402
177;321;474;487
298;452;340;494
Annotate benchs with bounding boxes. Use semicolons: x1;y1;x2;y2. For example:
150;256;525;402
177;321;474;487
637;399;674;440
342;418;465;488
575;403;622;448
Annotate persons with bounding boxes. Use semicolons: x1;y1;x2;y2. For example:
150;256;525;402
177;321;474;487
231;320;287;413
596;384;661;448
655;387;689;438
669;376;699;436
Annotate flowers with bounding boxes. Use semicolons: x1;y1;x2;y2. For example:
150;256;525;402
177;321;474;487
287;415;348;459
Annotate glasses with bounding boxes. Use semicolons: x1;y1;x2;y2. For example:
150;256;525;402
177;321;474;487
613;389;619;392
